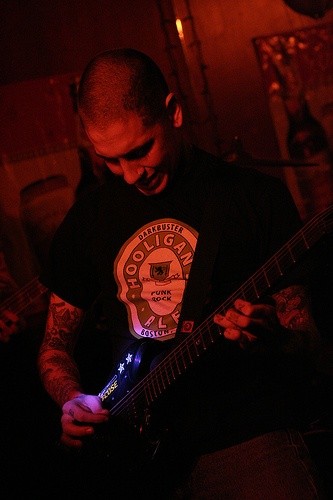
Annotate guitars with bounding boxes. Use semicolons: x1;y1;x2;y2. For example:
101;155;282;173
96;204;332;427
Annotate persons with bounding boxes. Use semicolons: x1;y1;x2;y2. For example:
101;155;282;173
35;49;332;472
